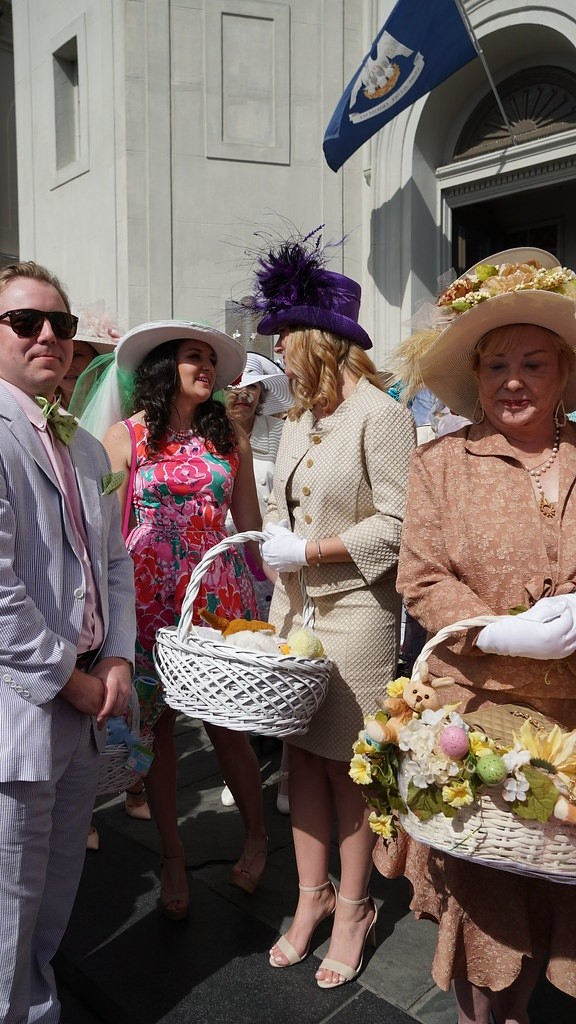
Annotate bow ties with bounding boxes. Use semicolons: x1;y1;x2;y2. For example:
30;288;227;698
35;394;79;447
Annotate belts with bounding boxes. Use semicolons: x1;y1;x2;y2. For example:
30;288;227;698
74;651;97;669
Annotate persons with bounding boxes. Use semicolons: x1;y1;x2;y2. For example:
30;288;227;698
55;309;150;849
371;246;576;1024
103;321;269;918
0;260;137;1024
220;348;295;813
390;382;473;447
233;241;418;989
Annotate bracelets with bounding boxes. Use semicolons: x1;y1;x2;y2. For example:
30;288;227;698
316;540;322;566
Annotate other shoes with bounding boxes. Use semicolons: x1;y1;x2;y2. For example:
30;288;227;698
221;781;236;806
276;782;292;815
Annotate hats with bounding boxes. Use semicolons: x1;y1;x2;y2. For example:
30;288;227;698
219;208;373;350
223;356;295;416
115;320;247;394
71;314;120;355
380;247;576;423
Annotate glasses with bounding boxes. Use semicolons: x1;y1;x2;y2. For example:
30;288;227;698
1;308;79;339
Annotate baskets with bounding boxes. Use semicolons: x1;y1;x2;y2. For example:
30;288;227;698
152;530;332;737
347;616;576;887
94;684;159;795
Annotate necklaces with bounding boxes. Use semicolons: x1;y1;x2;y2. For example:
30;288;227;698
525;423;559;518
167;426;191;437
248;432;252;437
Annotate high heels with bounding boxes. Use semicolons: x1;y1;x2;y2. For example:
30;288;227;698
267;879;338;968
125;789;152;820
85;826;98;851
160;852;189;920
315;892;378;988
229;837;270;892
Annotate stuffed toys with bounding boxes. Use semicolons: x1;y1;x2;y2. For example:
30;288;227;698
366;660;455;746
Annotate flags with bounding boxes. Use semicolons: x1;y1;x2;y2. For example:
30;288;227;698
323;0;479;173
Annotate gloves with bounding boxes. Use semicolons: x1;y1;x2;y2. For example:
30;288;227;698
475;592;576;661
258;522;309;573
